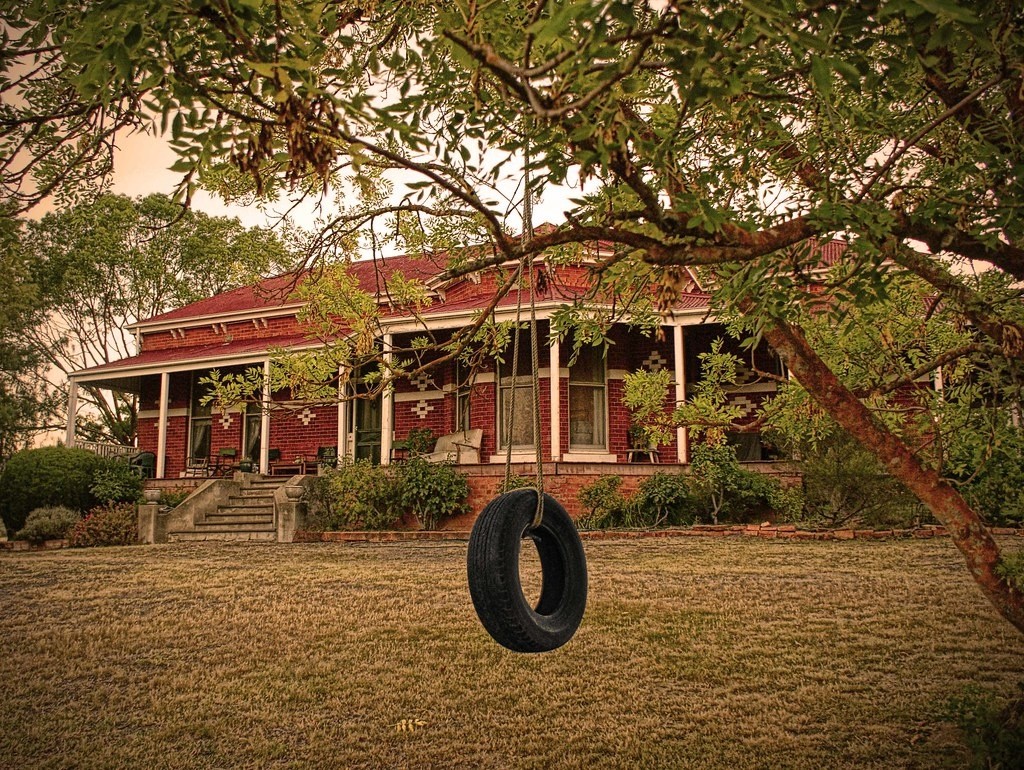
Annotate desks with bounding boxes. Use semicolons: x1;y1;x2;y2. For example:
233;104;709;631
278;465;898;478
627;449;661;463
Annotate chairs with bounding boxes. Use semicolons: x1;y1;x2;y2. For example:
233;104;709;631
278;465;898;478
389;441;410;466
303;446;338;475
130;452;156;477
206;448;235;477
184;454;217;476
268;448;280;476
272;464;304;475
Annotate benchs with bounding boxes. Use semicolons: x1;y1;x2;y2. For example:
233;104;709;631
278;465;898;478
417;429;484;464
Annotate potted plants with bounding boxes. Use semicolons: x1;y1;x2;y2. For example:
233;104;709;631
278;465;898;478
628;424;647;444
